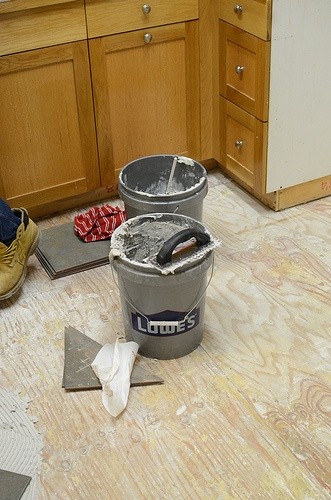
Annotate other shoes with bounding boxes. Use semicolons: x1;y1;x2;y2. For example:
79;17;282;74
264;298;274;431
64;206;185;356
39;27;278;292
1;206;42;301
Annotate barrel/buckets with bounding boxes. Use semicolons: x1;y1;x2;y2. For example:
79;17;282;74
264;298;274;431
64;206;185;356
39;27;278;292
119;155;208;222
109;213;214;359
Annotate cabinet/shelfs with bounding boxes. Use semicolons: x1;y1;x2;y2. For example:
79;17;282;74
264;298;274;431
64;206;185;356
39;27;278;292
212;0;331;212
0;0;216;222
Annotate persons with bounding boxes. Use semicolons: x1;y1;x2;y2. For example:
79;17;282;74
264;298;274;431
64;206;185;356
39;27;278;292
0;198;42;301
